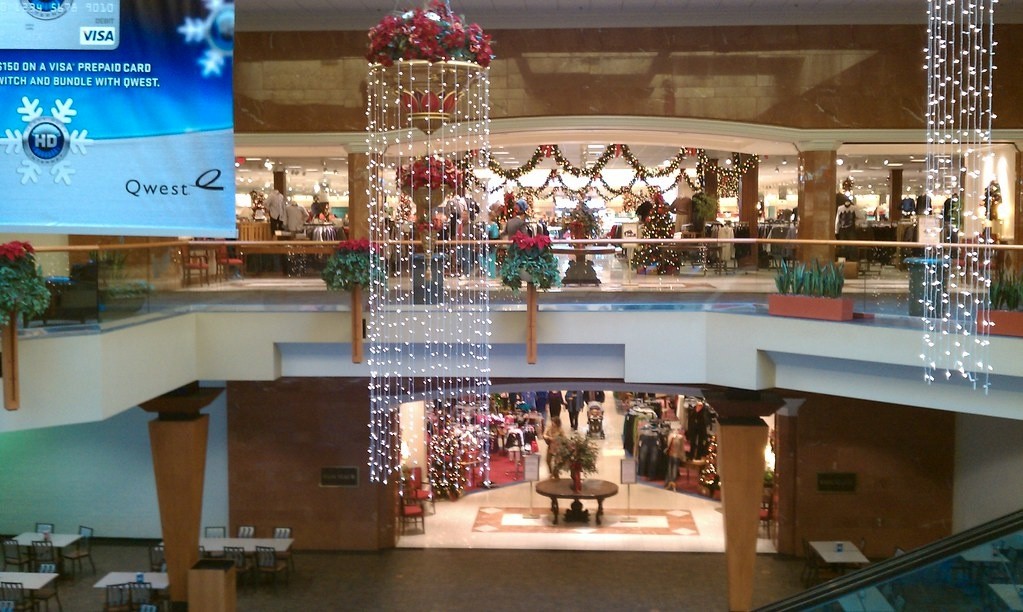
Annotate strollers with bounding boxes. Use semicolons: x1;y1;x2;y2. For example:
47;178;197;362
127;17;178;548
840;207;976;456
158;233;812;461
586;401;605;439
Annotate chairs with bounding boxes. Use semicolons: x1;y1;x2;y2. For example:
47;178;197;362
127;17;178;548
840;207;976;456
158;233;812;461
0;467;436;612
799;535;869;591
25;260;102;329
758;493;772;541
180;245;210;287
214;238;244;283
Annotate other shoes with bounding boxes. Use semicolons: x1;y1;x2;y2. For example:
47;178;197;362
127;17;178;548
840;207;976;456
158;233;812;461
571;427;574;431
574;430;578;434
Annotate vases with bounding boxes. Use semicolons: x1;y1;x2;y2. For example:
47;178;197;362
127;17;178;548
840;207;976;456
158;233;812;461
519;268;535;283
570;463;583;493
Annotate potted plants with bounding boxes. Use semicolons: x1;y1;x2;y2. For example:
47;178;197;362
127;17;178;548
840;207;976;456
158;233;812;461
90;251;155;316
976;263;1023;336
768;257;855;321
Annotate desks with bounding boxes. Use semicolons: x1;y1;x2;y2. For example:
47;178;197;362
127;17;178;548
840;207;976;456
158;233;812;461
809;540;870;577
682;459;707;486
92;571;170;604
459;460;484;488
154;537;295;569
0;571;60;612
535;478;619;525
668;245;707;278
3;532;84;575
548;244;616;264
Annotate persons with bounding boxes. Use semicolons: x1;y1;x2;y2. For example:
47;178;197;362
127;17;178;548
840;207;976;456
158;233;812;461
499;211;529;287
543;416;565;480
902;192;932;217
586;403;605;440
504;433;523;479
458;211;498;281
688;402;711;460
544;390;605;429
944;193;959;220
666;431;686;493
264;190;309;232
835;201;860;261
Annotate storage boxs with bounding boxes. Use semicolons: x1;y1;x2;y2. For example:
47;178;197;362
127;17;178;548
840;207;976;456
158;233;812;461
464;450;480;461
507;446;521;463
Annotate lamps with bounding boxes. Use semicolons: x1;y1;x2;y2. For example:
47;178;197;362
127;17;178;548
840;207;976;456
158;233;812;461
726;158;731;164
881;158;889;165
836;158;844;165
775;167;779;173
240;157;339;183
780;155;787;165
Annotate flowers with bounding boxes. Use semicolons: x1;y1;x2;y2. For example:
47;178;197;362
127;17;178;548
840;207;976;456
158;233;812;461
499;229;563;298
321;238;388;296
0;239;53;331
363;0;495;68
